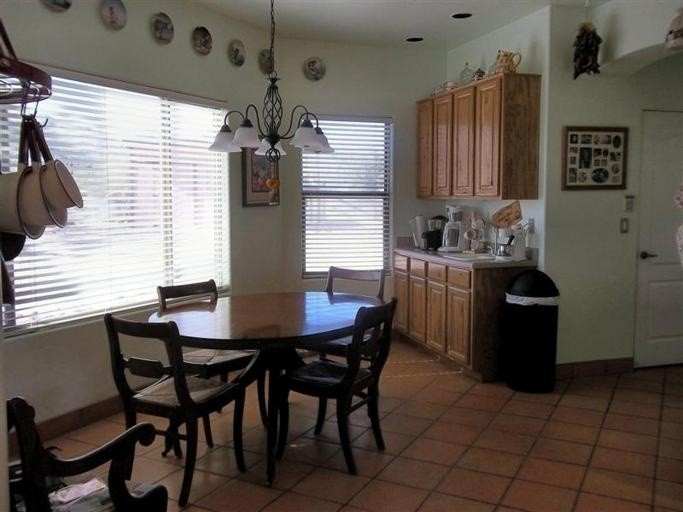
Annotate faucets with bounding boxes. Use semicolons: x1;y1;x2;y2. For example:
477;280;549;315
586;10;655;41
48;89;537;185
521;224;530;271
494;227;498;255
486;245;493;255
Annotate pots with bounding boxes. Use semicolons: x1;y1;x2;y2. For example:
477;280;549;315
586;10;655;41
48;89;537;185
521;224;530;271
0;114;85;307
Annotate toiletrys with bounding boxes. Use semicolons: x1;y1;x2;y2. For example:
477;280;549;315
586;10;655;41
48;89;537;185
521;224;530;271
415;215;426;244
412;231;420;249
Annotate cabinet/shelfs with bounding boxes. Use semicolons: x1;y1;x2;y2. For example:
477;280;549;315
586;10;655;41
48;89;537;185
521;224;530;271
414;92;451;202
392;252;425;344
452;73;540;201
424;263;537;376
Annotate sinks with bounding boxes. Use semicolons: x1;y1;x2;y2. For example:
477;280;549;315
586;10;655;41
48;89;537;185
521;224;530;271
443;253;496;261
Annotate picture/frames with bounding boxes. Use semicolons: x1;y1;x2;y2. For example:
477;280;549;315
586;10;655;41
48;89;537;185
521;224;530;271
561;125;629;190
239;134;280;207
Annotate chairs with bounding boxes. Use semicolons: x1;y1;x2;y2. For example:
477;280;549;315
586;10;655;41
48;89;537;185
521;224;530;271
155;278;270;449
317;265;385;401
6;395;168;512
103;312;249;508
272;296;398;474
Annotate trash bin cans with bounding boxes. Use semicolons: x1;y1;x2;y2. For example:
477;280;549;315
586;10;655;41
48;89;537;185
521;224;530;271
498;267;561;395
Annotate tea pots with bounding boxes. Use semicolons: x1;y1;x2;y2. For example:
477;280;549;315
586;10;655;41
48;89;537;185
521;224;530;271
495;47;520;75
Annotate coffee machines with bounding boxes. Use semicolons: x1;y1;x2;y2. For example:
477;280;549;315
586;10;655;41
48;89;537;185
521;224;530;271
408;204;471;252
494;224;527;261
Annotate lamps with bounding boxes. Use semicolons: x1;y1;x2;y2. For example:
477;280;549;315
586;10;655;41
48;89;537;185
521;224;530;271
208;1;335;163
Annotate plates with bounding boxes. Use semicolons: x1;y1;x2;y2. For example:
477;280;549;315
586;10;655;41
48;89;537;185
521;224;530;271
38;0;72;15
150;11;174;46
191;25;213;55
227;40;247;68
301;57;326;80
98;0;127;33
257;49;274;76
